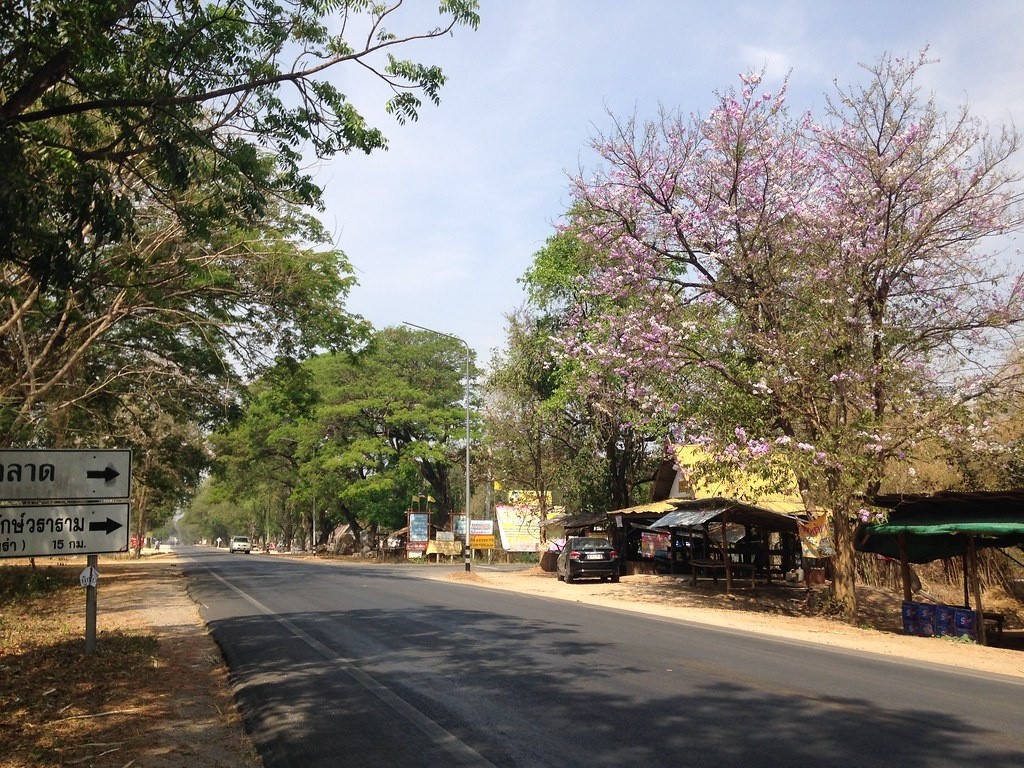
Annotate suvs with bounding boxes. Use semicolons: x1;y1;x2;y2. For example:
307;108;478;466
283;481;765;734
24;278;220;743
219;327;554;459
229;535;252;554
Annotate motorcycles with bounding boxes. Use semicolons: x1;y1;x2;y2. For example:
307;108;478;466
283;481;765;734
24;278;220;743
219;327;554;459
154;542;161;550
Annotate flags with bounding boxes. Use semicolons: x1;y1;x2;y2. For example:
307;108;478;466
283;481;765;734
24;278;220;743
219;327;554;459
428;496;435;503
413;496;420;501
419;492;426;498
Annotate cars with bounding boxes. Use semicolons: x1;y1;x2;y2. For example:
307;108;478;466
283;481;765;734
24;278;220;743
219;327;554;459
270;542;288;550
252;544;261;551
554;537;619;584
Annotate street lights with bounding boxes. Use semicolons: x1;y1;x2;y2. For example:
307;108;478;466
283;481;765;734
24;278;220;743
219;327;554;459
404;322;471;570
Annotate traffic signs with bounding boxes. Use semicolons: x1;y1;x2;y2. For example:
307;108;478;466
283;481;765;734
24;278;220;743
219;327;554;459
0;449;131;557
